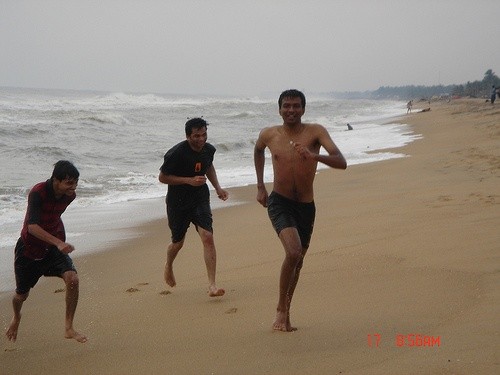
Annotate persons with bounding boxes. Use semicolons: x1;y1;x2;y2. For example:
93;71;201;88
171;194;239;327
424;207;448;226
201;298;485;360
6;161;88;343
254;89;347;332
347;123;353;129
491;85;497;104
159;118;228;296
418;107;430;113
407;101;412;114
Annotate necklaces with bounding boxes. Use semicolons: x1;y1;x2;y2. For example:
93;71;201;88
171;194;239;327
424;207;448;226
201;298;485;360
282;125;304;145
